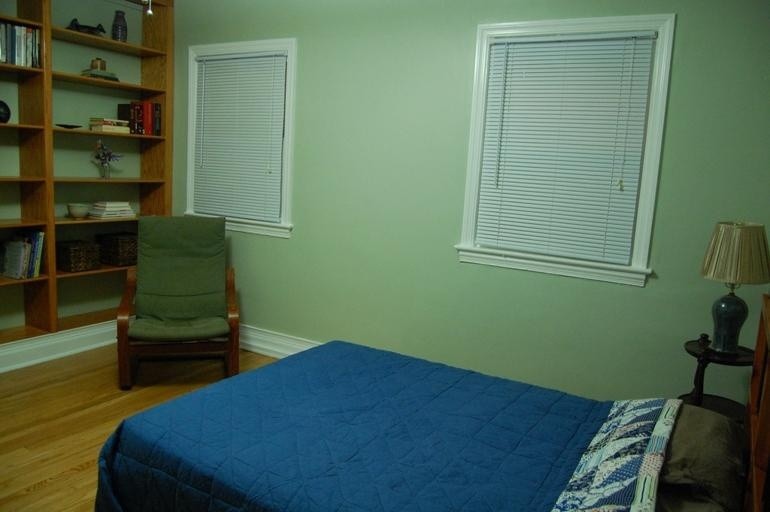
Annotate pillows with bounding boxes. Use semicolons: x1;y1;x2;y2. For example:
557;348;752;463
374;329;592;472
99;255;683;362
661;401;749;512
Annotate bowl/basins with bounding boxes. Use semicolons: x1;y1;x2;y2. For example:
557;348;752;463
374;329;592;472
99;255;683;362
68;202;90;221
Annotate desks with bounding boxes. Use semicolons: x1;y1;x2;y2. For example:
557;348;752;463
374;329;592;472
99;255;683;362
678;335;754;424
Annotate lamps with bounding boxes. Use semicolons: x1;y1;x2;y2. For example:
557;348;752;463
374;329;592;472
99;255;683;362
697;221;769;357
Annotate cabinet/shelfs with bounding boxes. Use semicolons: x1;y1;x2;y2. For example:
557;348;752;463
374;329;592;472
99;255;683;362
1;0;174;346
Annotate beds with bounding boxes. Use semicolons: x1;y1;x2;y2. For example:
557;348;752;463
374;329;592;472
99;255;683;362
96;294;770;512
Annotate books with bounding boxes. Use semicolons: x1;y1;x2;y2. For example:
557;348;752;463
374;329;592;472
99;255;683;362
86;101;162;137
89;199;139;221
0;22;44;68
1;231;45;280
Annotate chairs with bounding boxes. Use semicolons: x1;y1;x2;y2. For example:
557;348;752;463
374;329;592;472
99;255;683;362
116;214;241;392
